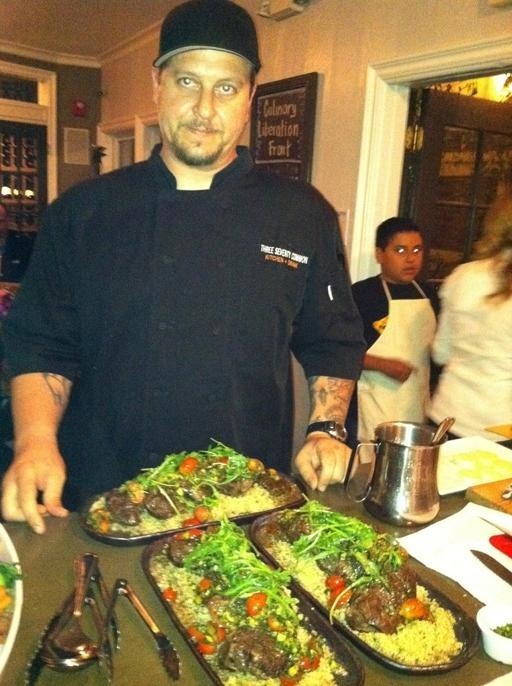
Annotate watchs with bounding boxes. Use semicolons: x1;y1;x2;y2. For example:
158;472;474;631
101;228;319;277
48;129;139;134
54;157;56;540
304;419;351;445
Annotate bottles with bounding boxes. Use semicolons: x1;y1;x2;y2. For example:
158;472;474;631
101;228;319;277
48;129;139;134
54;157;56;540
0;133;45;231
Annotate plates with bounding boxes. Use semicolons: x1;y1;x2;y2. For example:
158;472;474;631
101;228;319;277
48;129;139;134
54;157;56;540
420;435;512;497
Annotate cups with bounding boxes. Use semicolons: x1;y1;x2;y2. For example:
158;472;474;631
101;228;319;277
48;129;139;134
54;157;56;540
344;418;450;531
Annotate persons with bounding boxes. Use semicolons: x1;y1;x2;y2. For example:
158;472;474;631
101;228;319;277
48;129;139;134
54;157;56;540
348;216;441;473
1;0;362;538
423;181;512;447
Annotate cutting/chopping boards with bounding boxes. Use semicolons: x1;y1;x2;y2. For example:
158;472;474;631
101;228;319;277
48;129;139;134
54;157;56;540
464;478;512;512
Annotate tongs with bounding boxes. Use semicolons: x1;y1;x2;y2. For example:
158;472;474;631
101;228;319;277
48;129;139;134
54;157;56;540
21;549;184;682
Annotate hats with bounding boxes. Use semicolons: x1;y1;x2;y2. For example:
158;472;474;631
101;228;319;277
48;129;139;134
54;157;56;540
152;0;262;68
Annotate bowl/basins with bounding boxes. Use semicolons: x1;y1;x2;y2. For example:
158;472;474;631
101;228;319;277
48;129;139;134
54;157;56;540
477;597;512;665
